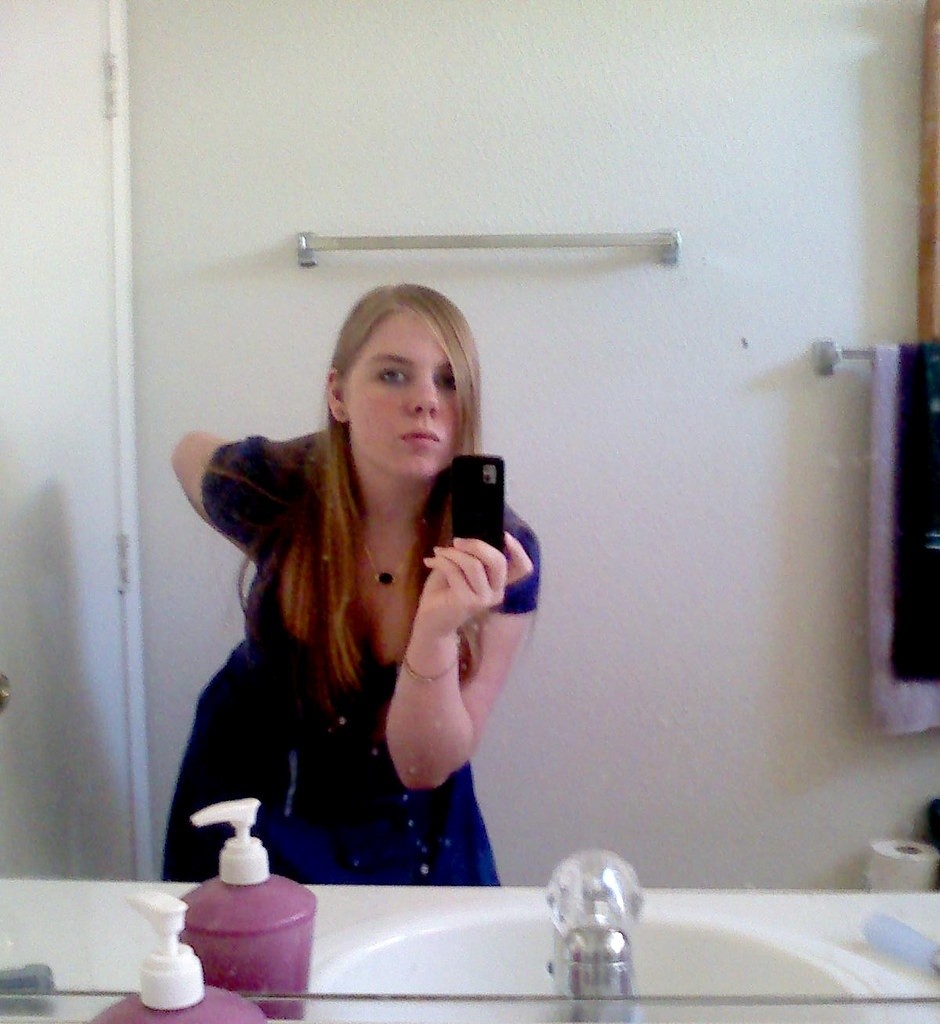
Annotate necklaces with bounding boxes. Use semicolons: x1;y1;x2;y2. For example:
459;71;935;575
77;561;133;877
358;517;424;586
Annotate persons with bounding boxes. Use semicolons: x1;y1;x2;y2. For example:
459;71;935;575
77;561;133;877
162;283;542;886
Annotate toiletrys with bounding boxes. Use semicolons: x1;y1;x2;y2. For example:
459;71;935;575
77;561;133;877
864;912;940;976
182;798;315;996
91;890;265;1023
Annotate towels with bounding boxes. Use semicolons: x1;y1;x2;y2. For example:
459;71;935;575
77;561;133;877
871;339;940;734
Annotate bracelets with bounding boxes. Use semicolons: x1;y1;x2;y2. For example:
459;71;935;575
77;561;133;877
401;657;455;683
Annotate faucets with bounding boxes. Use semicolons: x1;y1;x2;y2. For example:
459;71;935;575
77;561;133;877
545;846;645;997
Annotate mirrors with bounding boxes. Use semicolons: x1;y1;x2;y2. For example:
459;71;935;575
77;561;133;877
0;0;940;1003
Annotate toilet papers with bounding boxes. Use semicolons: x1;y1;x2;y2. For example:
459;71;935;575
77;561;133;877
871;838;939;893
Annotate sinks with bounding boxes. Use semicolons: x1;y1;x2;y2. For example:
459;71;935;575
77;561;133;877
319;890;915;998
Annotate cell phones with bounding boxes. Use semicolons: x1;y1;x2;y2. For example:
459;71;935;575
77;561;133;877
448;454;505;553
